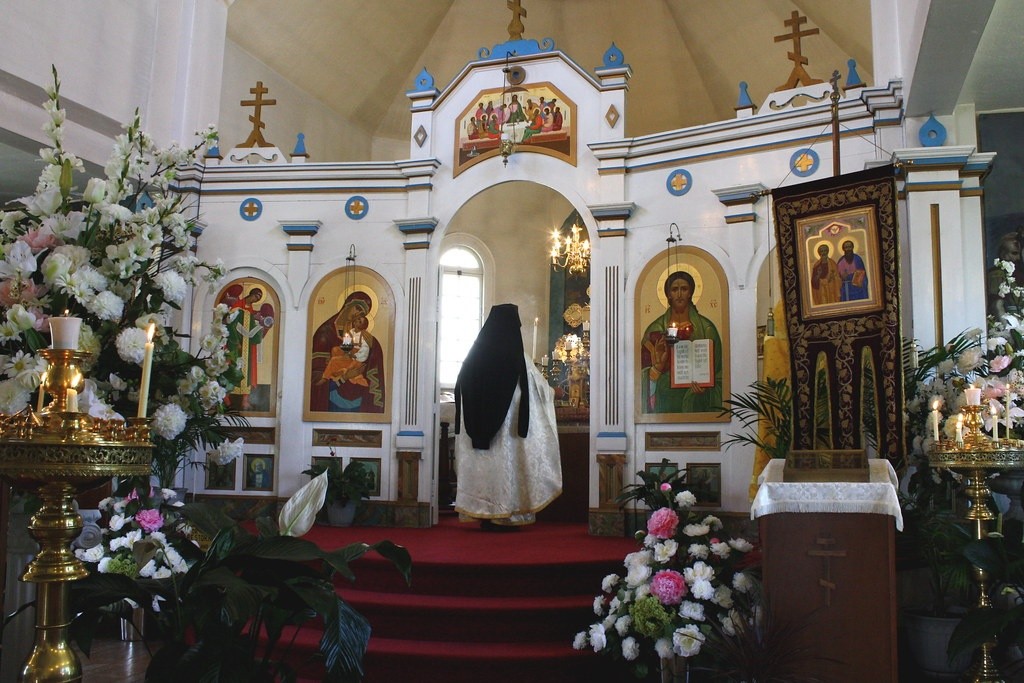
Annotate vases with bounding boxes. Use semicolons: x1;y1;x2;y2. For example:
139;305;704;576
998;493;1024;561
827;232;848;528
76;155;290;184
121;607;143;641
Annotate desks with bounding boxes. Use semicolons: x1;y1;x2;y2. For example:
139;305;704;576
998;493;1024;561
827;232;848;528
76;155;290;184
438;402;590;498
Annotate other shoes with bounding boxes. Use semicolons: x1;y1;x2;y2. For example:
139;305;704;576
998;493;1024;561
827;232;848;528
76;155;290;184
480;519;521;532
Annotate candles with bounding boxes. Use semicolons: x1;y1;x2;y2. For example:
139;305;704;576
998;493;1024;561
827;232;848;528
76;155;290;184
341;331;354;347
531;317;538;361
582;321;590;332
932;382;1010;443
36;309;155;417
540;354;550;366
563;340;571;350
667;320;678;337
552;349;560;359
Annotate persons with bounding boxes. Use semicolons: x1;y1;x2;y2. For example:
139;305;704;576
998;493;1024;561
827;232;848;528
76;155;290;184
451;303;564;531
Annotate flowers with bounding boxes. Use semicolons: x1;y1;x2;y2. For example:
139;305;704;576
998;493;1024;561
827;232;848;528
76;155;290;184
70;478;199;580
0;63;246;469
906;258;1024;484
571;483;761;683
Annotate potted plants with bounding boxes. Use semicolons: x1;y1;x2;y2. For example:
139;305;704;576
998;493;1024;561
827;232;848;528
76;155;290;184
302;462;376;526
898;477;978;678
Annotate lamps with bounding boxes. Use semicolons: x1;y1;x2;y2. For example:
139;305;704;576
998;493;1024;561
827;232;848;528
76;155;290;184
551;224;594;278
